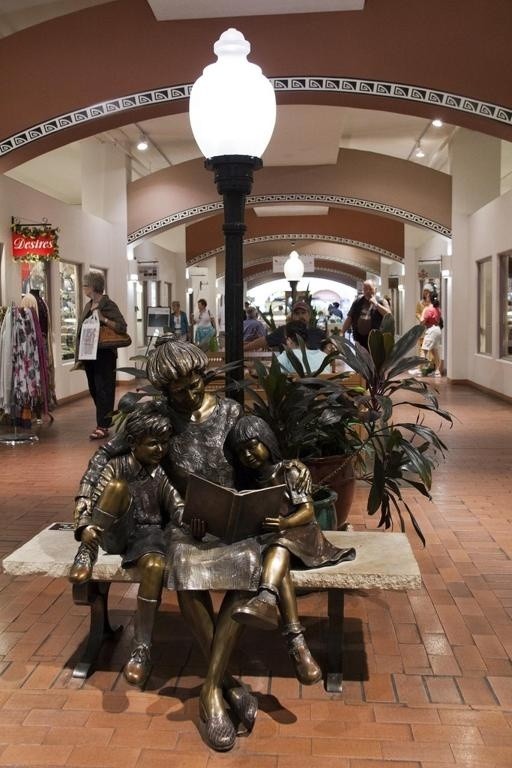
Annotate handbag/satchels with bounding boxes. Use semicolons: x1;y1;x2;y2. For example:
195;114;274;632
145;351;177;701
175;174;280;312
98;302;132;350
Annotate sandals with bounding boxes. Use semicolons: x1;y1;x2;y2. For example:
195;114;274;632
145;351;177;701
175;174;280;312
89;428;109;440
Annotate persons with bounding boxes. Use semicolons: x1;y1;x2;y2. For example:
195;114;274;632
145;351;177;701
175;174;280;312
245;302;333;353
73;341;273;750
416;283;433;370
406;291;443;377
340;280;393;349
243;308;268;343
75;272;129;439
328;303;343;319
70;404;176;683
169;301;188;343
227;414;355;686
190;299;216;347
269;320;333;376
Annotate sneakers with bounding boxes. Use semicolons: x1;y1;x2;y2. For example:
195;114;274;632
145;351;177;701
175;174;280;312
427;369;442;379
408;368;424;378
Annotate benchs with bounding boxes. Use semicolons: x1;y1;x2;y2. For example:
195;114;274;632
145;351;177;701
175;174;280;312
2;520;425;694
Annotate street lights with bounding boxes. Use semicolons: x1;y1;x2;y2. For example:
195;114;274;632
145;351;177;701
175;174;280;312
285;249;304;309
190;28;276;414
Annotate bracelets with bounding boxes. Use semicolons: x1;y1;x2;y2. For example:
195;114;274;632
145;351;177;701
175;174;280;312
104;318;109;323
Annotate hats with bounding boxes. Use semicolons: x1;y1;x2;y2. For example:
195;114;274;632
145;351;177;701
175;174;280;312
292;301;309;311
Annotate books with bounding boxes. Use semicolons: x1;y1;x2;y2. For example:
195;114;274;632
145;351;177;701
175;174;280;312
182;470;288;544
